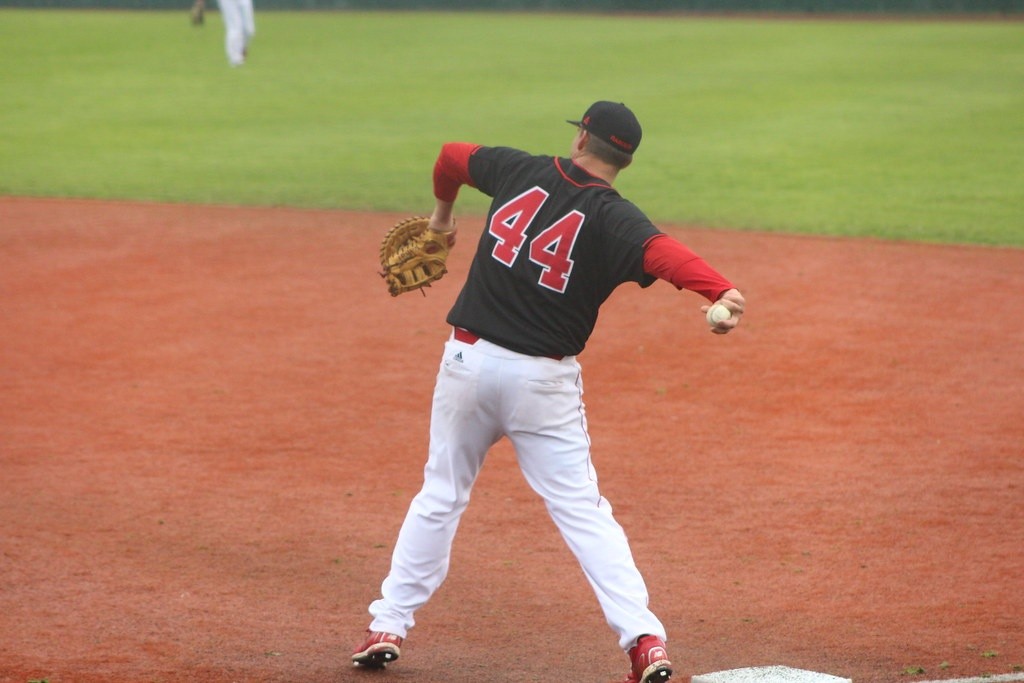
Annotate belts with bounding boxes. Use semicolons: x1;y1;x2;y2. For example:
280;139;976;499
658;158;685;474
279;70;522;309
454;327;565;361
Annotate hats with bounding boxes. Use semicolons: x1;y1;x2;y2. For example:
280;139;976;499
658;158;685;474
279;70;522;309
566;101;643;155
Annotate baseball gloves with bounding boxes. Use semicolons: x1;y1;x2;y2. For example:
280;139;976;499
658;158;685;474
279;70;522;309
378;214;460;297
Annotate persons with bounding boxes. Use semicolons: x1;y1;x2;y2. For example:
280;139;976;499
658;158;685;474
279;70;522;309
352;100;746;683
190;0;255;66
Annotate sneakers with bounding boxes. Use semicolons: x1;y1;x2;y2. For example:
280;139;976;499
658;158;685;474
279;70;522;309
351;628;403;667
625;636;673;683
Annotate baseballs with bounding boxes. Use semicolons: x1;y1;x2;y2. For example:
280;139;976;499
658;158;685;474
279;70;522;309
706;304;731;330
